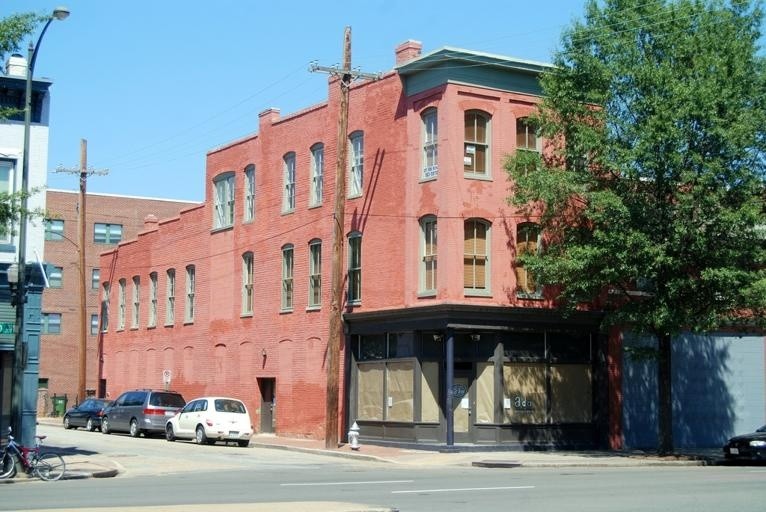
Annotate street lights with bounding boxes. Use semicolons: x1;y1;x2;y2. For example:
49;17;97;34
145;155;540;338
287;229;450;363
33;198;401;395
10;5;70;471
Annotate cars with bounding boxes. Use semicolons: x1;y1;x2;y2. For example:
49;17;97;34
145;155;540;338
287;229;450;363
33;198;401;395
61;398;115;430
164;395;254;448
724;424;766;467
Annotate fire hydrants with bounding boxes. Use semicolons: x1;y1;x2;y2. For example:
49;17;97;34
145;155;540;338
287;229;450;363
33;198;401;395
348;421;361;450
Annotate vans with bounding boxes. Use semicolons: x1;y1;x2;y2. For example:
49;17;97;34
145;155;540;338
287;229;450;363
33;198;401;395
101;389;186;439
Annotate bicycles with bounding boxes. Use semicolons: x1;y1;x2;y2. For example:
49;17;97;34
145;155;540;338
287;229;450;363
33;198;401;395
0;425;64;480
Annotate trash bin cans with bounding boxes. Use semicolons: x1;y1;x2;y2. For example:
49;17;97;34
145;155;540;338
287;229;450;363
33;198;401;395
50;396;68;417
